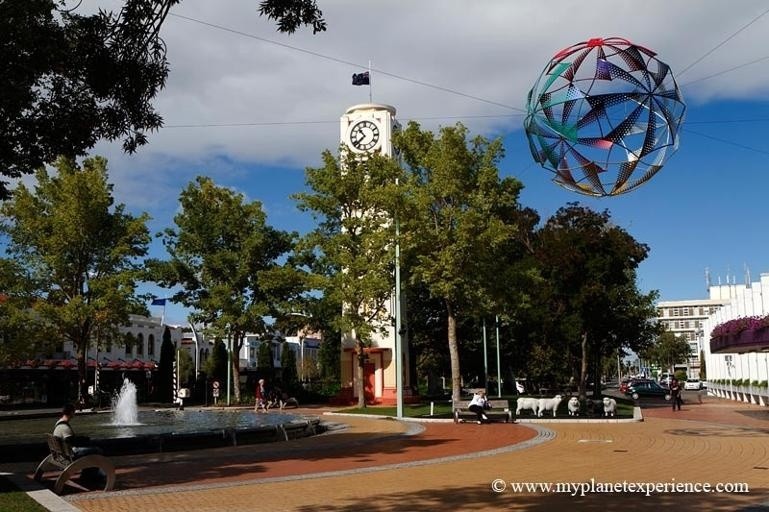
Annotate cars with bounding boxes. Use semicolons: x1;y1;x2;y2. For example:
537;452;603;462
619;378;632;395
626;378;672;402
684;379;700;390
661;373;674;384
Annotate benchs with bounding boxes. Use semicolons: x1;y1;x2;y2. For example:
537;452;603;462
32;433;118;495
452;398;513;425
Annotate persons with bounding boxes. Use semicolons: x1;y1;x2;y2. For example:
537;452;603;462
53;404;105;483
669;378;681;411
255;379;268;411
468;391;489;424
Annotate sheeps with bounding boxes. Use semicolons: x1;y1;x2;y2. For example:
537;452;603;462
538;395;562;417
568;397;581;416
603;398;617;417
516;397;540;415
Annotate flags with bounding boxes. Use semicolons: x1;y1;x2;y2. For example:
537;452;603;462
151;299;165;305
351;72;369;84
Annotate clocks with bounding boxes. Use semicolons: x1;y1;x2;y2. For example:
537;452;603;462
341;112;383;154
